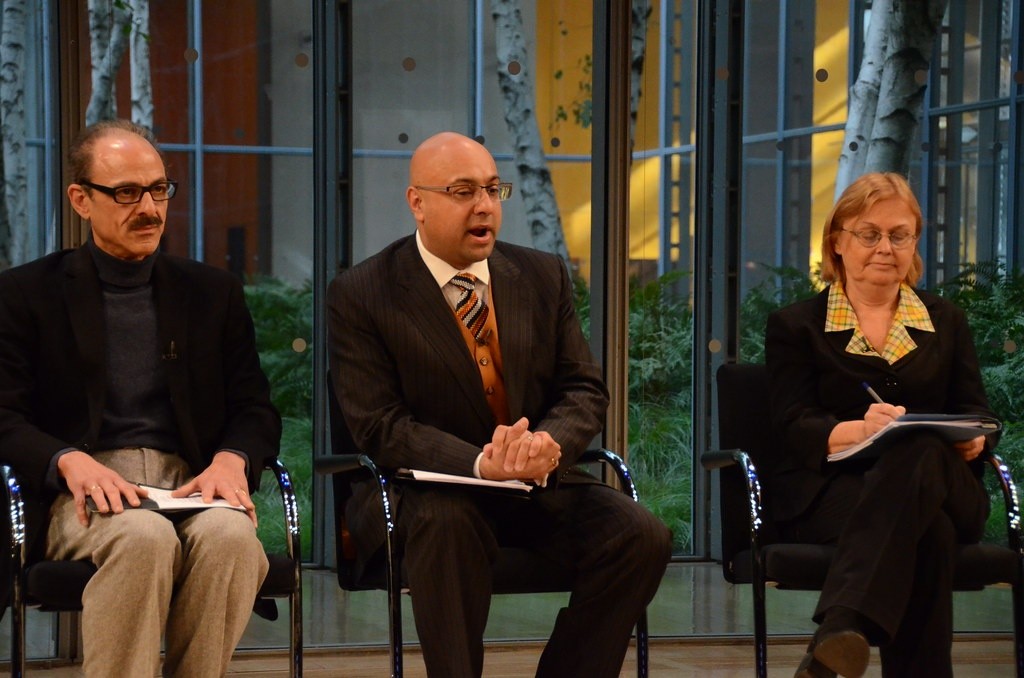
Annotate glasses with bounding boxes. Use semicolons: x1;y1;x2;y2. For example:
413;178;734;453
839;228;916;249
74;178;178;204
416;183;512;203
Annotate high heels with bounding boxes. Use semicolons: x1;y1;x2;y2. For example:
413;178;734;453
795;609;869;678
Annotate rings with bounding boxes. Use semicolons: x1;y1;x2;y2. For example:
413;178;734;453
552;458;557;466
528;436;532;441
92;486;100;489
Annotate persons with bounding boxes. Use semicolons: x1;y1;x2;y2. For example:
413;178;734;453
767;172;1004;678
0;121;284;678
326;132;675;678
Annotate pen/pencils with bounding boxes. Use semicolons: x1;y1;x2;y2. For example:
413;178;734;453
861;382;884;404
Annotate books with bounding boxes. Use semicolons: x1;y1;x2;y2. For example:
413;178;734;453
826;414;1002;462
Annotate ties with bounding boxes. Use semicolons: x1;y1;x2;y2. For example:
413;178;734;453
446;272;489;339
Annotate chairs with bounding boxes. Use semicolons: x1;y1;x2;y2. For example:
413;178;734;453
317;366;650;678
703;363;1024;678
1;450;302;678
